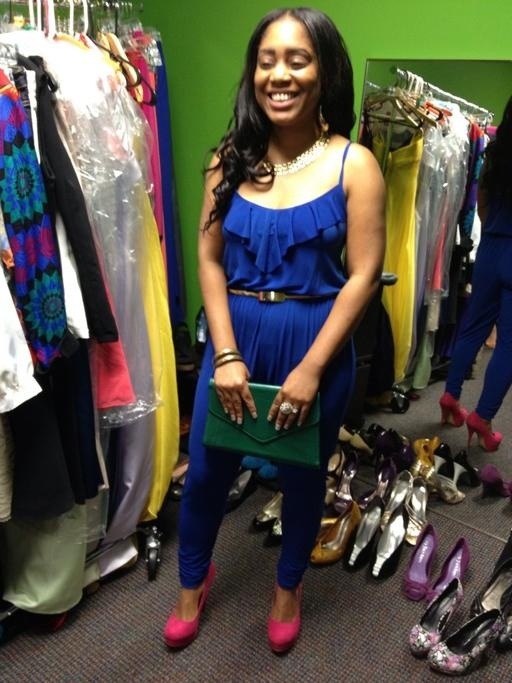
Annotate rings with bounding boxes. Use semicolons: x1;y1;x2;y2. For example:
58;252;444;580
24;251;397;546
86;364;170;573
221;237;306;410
292;408;300;416
279;401;293;415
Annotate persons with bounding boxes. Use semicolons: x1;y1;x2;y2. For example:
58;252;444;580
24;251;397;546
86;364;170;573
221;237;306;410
162;5;386;654
439;94;511;452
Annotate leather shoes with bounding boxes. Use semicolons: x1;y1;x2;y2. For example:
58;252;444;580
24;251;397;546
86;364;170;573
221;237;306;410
169;457;192;502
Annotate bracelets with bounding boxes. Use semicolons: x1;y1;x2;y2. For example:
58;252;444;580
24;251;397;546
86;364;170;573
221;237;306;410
214;348;240;362
213;354;243;369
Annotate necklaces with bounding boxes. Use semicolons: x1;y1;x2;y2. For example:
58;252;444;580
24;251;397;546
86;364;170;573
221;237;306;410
262;121;330;175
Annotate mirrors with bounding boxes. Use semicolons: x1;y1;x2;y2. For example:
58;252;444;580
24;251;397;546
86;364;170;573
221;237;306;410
355;58;512;545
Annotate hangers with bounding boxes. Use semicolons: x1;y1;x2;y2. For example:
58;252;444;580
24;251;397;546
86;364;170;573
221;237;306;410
362;65;494;137
0;0;162;106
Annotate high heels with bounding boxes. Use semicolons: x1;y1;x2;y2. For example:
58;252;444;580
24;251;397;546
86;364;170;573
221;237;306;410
469;558;512;618
491;610;512;651
428;608;504;675
224;468;257;502
253;487;285;531
267;581;301;655
464;410;507;455
264;516;283;549
339;424;480;503
481;464;511;498
308;450;428;581
410;577;464;659
436;389;467;430
403;524;438;600
429;536;471;599
162;556;218;650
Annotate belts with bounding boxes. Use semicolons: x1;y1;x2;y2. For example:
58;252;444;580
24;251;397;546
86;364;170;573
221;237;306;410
224;286;333;304
